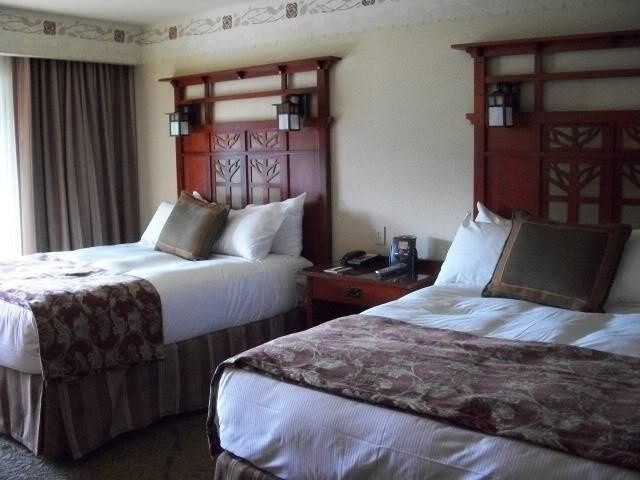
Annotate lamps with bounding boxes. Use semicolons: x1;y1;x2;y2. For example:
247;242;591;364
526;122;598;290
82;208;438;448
480;82;521;128
271;94;310;131
164;104;201;137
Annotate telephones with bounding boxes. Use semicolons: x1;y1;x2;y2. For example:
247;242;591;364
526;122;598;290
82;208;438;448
342;250;381;269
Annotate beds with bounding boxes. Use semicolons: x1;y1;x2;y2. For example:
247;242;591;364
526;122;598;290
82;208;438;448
210;30;639;479
1;57;342;461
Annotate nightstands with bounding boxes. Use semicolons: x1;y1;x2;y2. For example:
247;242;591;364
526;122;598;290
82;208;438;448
296;256;444;330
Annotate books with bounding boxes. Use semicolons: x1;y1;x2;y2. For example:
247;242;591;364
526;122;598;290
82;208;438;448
323;266;352;274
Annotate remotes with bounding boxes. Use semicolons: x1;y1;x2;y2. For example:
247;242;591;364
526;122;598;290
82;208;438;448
373;262;408;278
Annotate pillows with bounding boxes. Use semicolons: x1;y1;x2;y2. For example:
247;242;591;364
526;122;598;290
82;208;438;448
138;190;307;260
433;201;640;313
